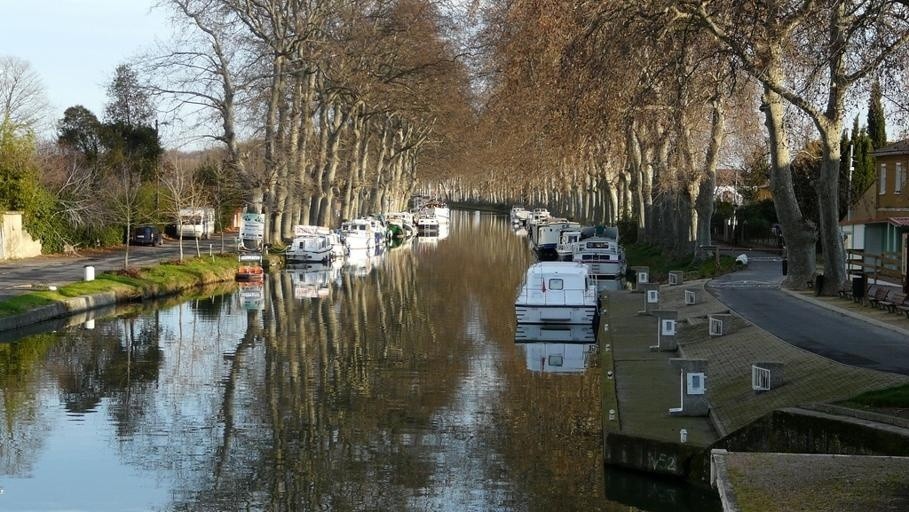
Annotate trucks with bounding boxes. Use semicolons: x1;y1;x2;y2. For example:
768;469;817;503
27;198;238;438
176;207;215;240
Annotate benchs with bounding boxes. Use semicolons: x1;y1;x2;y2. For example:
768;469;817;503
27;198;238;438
838;282;908;316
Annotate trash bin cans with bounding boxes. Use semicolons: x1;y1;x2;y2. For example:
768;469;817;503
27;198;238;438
84;265;95;281
853;271;867;297
782;256;788;275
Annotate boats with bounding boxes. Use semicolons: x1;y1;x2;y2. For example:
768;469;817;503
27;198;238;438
285;195;449;273
510;205;626;325
513;325;598;374
285;271;341;298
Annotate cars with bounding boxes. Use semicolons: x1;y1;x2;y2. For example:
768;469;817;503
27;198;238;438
133;226;163;246
